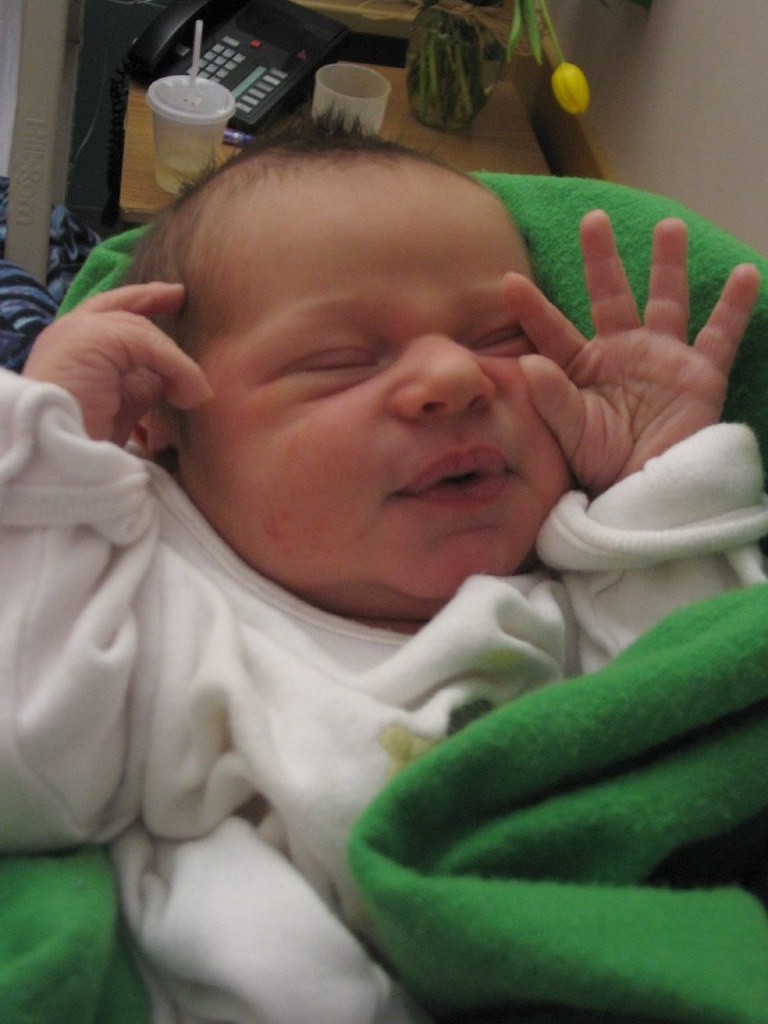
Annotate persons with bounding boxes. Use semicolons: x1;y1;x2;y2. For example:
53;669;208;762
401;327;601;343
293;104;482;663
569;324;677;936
1;98;768;1023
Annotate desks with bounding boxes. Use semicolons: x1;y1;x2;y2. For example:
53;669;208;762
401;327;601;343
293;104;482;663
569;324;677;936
121;0;552;224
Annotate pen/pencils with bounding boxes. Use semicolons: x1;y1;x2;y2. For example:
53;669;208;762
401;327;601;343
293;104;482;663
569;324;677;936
221;127;252;147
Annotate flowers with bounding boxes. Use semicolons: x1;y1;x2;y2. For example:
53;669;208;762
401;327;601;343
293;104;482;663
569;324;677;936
409;0;589;126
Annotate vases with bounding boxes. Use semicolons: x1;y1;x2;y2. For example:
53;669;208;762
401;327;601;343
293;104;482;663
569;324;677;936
408;0;514;131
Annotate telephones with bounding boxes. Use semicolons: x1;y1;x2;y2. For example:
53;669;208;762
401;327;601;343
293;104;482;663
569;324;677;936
124;0;354;136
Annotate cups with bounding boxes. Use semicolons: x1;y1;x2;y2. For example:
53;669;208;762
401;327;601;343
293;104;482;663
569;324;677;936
310;61;391;135
145;74;237;195
